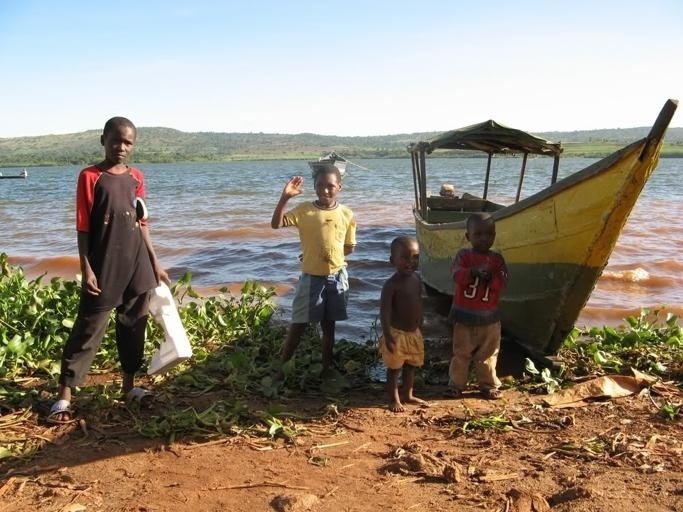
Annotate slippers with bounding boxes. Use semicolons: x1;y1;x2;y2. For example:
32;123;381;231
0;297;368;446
46;400;76;424
126;387;155;406
442;388;461;398
484;388;503;399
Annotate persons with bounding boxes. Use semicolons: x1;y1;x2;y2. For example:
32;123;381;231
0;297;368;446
381;236;429;412
47;116;170;424
441;212;507;399
270;164;356;371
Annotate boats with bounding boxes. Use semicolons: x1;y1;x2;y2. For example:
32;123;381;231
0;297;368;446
406;97;680;358
307;150;347;176
